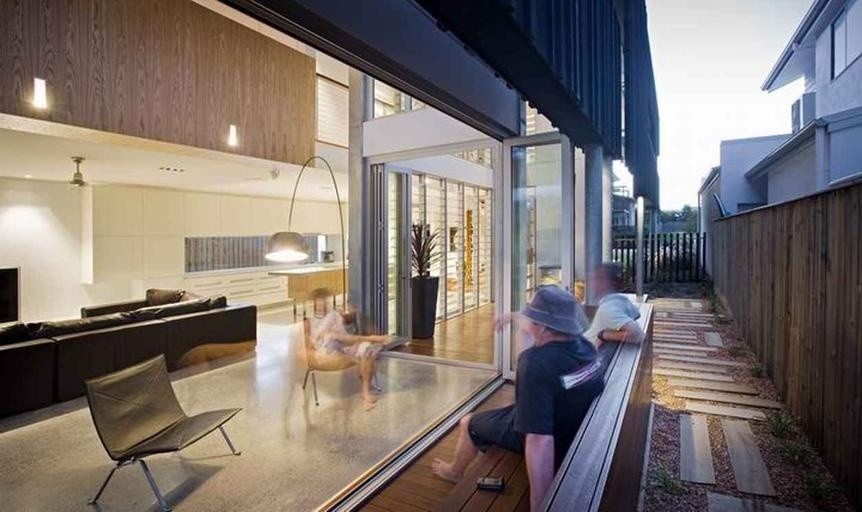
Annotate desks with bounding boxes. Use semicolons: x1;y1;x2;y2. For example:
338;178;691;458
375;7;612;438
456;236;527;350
268;261;348;320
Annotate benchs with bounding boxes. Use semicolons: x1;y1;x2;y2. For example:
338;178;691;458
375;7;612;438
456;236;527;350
436;303;654;512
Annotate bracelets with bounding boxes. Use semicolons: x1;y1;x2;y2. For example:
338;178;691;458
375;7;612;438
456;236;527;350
597;330;608;342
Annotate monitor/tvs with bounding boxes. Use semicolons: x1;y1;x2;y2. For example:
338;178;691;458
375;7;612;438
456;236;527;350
0;266;21;324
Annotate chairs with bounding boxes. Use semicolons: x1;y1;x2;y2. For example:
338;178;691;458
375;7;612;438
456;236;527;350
302;319;381;405
82;354;243;511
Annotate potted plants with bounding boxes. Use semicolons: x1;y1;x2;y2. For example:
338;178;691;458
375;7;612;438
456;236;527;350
409;222;440;340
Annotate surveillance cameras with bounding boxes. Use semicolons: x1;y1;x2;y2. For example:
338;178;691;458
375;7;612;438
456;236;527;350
270;170;280;181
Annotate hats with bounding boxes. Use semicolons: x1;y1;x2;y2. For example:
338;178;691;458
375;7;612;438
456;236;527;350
518;286;591;335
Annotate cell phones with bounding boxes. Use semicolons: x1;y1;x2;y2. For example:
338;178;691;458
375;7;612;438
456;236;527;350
477;476;504;491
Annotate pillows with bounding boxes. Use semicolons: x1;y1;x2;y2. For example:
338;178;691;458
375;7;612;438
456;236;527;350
146;288;185;306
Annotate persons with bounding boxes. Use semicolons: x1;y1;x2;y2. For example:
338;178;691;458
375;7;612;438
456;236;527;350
432;285;605;512
580;261;645;350
304;287;394;404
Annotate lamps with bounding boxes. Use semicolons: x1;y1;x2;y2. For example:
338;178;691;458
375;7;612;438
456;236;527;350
69;159;86;188
265;156;358;336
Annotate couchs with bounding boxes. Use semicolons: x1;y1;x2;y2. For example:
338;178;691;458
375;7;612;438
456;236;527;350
0;291;256;422
